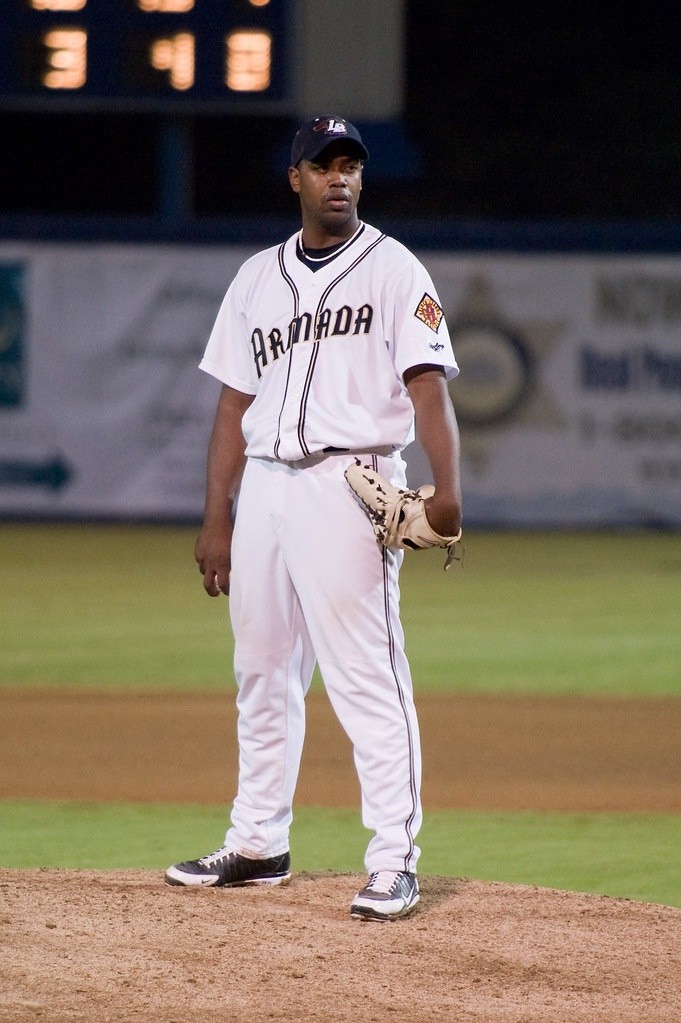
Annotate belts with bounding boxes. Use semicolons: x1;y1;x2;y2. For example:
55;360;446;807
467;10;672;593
323;445;350;453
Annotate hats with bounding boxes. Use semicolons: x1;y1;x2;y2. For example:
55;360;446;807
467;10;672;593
292;114;370;168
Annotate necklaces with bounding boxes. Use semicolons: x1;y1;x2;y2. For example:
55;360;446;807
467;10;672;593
298;219;363;262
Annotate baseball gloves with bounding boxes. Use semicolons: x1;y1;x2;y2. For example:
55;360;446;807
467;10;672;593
342;459;463;553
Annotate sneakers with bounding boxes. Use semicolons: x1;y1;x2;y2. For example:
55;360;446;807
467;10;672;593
164;844;291;888
350;869;422;922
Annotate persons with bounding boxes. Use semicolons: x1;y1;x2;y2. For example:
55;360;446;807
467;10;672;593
164;114;463;923
